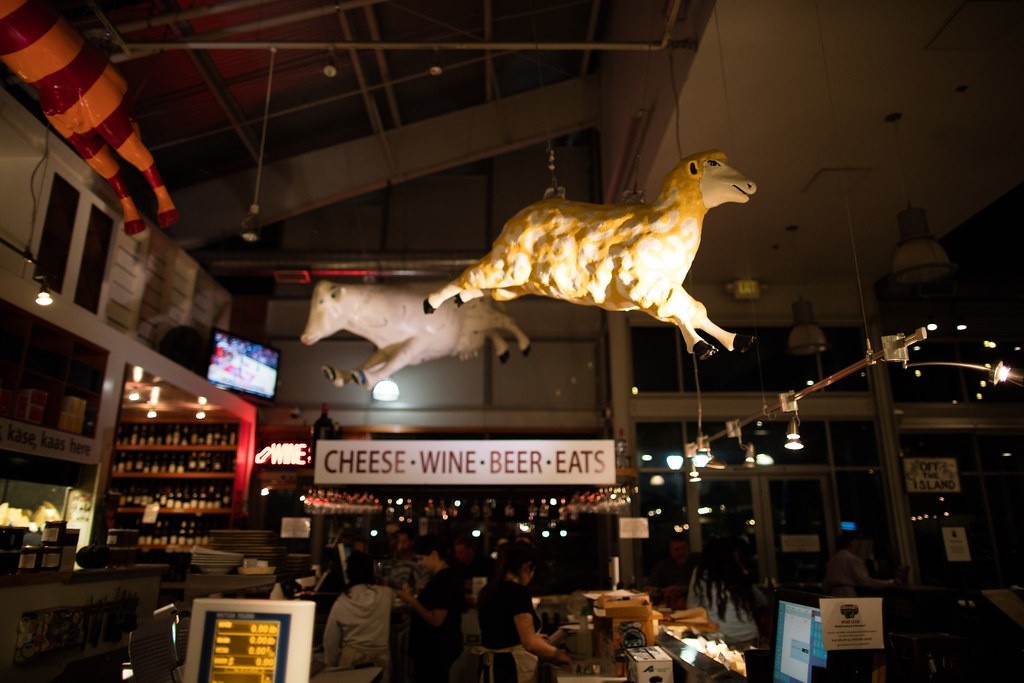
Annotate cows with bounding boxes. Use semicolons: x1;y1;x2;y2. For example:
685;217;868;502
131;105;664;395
301;280;533;388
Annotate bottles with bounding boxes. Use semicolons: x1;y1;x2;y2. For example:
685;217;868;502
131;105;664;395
312;405;338;464
407;557;418;597
112;449;235;474
134;518;210;546
541;610;562;635
119;484;231;510
116;423;236;446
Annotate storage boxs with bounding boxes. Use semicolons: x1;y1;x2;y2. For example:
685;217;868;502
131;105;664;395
594;591;675;683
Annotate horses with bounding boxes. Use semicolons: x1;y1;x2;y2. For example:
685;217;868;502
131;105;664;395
0;0;181;237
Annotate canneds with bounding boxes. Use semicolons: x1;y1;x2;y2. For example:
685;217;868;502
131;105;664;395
17;520;68;573
102;528;140;569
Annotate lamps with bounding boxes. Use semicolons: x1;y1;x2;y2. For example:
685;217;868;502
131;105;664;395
429;53;443;77
34;275;53;305
240;204;261;242
638;112;1009;484
323;54;337;77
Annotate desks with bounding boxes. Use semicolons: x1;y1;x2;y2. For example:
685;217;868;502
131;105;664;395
856;578;946;634
309;662;385;683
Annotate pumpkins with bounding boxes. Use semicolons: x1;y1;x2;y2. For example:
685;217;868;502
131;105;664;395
76;538;112;570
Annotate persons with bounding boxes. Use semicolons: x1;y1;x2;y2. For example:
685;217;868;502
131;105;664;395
650;535;698;603
382;529;419;590
686;533;760;683
394;534;464;683
312;528;396;683
452;537;492;577
477;539;572;683
833;531;871;597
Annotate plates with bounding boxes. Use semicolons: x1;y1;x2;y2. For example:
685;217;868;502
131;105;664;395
212;530;313;556
244;556;312;574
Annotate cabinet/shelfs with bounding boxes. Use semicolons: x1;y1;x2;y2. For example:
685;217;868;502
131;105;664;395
93;420;252;616
0;297;109;438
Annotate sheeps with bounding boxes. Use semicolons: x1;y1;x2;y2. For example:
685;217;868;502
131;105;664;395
423;149;759;360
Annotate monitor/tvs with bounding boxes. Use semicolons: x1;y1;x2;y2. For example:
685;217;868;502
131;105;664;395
770;586;874;683
200;327;283;402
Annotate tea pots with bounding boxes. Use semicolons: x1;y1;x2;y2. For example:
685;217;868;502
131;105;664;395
76;544;112;569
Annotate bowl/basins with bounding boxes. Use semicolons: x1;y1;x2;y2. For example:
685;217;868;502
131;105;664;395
190;546;244;575
237;566;276;574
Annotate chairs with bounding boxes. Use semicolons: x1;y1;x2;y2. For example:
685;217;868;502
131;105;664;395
128;617;190;683
